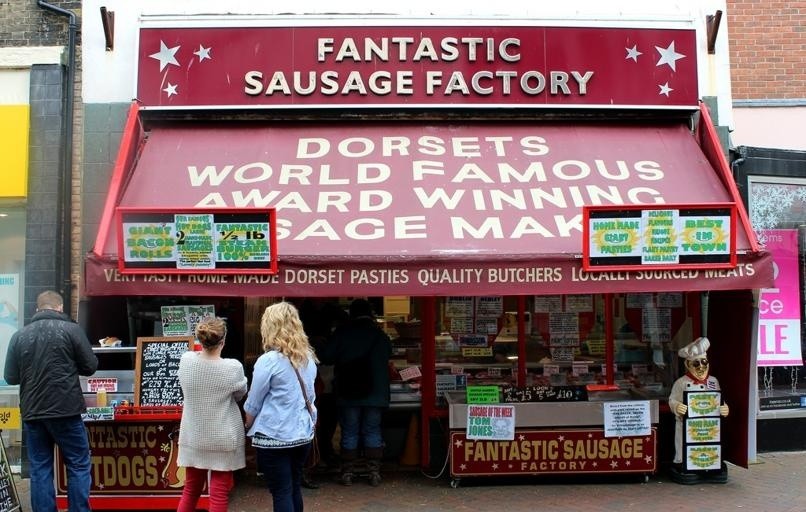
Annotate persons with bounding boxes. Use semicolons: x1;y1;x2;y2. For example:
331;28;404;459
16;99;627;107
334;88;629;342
243;301;392;512
4;289;98;512
176;316;248;512
668;336;729;486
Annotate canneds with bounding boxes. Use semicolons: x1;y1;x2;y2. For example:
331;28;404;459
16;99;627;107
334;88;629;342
109;400;134;415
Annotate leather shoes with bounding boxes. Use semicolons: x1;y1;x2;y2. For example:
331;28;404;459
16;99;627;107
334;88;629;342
300;471;318;489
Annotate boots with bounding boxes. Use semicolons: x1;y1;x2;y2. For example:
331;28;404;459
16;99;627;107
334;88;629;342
364;447;382;487
334;448;353;487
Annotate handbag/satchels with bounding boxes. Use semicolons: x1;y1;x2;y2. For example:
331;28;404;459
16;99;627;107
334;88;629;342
303;431;321;469
